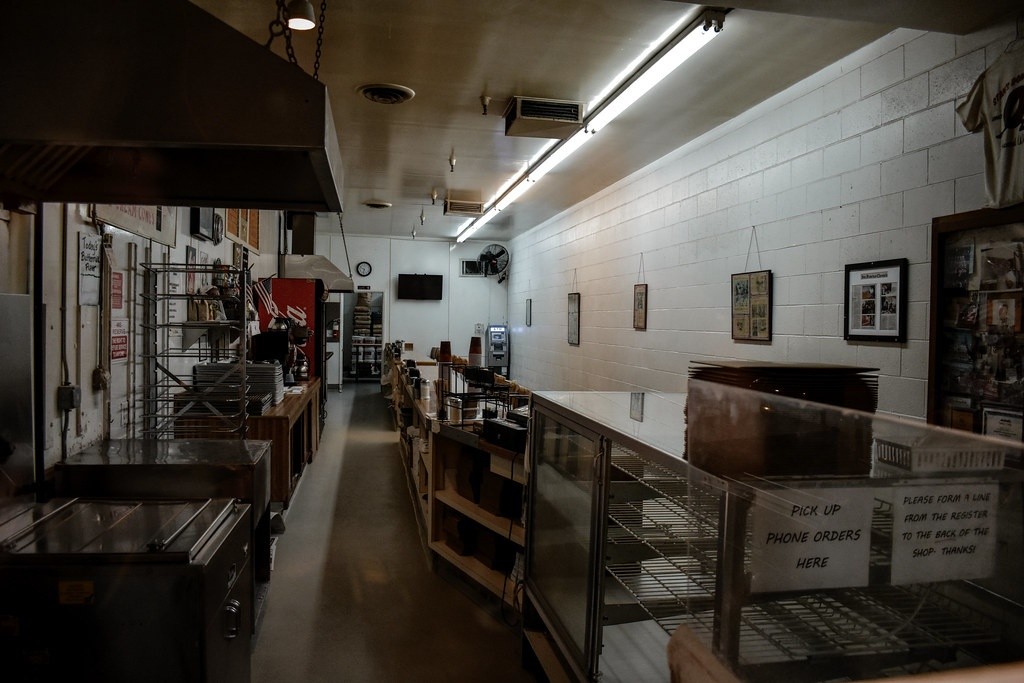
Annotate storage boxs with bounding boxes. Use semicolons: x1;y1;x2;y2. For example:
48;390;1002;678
190;207;214;240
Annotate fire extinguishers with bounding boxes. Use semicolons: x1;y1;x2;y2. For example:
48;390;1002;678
332;318;340;341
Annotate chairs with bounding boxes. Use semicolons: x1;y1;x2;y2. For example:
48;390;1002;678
429;346;529;407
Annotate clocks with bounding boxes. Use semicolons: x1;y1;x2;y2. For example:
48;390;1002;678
356;261;371;276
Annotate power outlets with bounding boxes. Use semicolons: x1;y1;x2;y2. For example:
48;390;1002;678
504;270;509;276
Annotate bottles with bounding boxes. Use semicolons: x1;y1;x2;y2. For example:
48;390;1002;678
420;380;431;399
449;398;462;425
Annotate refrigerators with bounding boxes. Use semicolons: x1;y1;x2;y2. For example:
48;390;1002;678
258;278;326;440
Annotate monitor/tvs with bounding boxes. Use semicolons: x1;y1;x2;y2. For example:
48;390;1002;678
398;274;443;301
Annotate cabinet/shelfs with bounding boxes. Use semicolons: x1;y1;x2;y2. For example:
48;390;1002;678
521;390;1024;681
233;242;248;269
58;438;271;586
138;263;251;437
430;405;534;623
1;496;257;682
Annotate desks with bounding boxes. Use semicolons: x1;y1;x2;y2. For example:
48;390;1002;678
384;343;436;431
173;378;322;505
351;341;381;381
394;365;516;552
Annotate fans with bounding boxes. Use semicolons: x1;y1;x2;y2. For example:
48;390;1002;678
477;243;509;283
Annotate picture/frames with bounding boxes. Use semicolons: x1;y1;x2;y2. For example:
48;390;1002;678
93;203;176;247
630;393;645;422
568;292;580;344
633;283;648;329
928;206;1023;470
525;298;531;325
731;270;772;340
844;258;909;344
